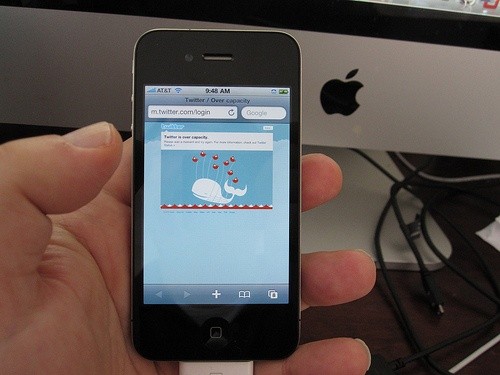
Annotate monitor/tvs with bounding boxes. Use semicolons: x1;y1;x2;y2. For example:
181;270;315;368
0;0;500;271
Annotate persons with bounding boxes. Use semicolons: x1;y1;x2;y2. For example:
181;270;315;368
0;121;377;375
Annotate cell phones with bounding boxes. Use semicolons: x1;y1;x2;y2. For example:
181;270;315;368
129;27;302;360
